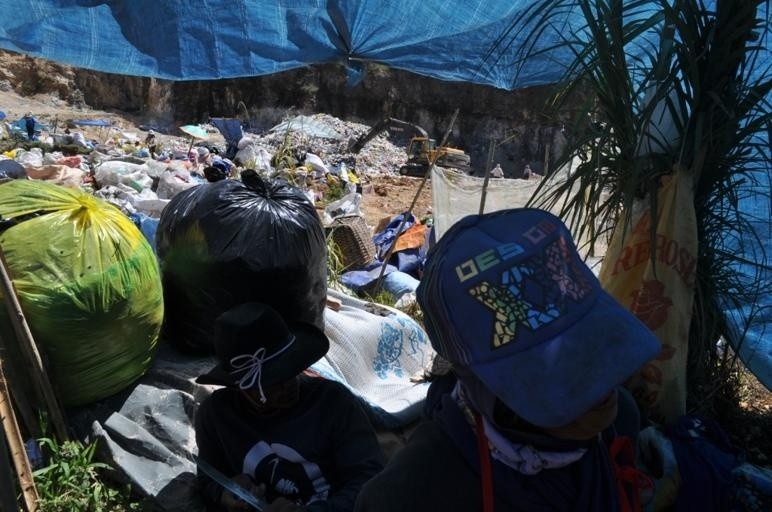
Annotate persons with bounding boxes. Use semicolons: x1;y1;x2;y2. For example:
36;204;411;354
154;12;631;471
524;164;532;179
350;209;662;512
191;299;384;512
491;164;504;179
25;112;38;140
144;129;157;157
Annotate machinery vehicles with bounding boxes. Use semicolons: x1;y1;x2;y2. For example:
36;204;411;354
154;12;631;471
349;115;472;179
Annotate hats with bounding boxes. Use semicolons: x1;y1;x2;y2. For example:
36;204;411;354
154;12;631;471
416;207;660;429
196;303;330;396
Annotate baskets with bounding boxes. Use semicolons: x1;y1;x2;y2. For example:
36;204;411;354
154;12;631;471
325;216;375;268
